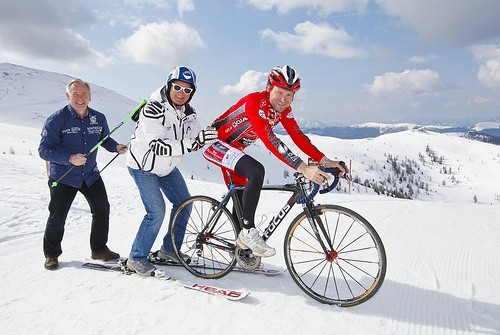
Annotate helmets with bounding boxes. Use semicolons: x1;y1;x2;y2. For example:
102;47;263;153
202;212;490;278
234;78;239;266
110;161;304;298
268;64;302;93
166;66;197;102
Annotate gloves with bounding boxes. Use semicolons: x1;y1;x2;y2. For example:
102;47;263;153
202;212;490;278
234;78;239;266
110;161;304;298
190;126;220;151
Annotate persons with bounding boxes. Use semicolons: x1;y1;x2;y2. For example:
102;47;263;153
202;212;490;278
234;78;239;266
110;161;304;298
203;65;349;271
124;67;218;277
37;78;128;271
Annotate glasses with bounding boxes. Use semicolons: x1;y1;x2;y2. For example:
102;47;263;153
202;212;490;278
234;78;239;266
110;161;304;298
273;89;293;99
171;82;194;95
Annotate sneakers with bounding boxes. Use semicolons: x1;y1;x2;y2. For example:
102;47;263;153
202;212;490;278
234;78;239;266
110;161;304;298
126;258;156;274
92;246;120;260
236;228;276;257
44;256;59;269
229;253;264;269
158;250;191;264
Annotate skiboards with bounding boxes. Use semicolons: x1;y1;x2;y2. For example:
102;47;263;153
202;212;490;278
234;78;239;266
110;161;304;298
82;257;288;301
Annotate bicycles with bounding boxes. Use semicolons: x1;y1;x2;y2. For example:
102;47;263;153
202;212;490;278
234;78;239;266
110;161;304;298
170;161;388;309
